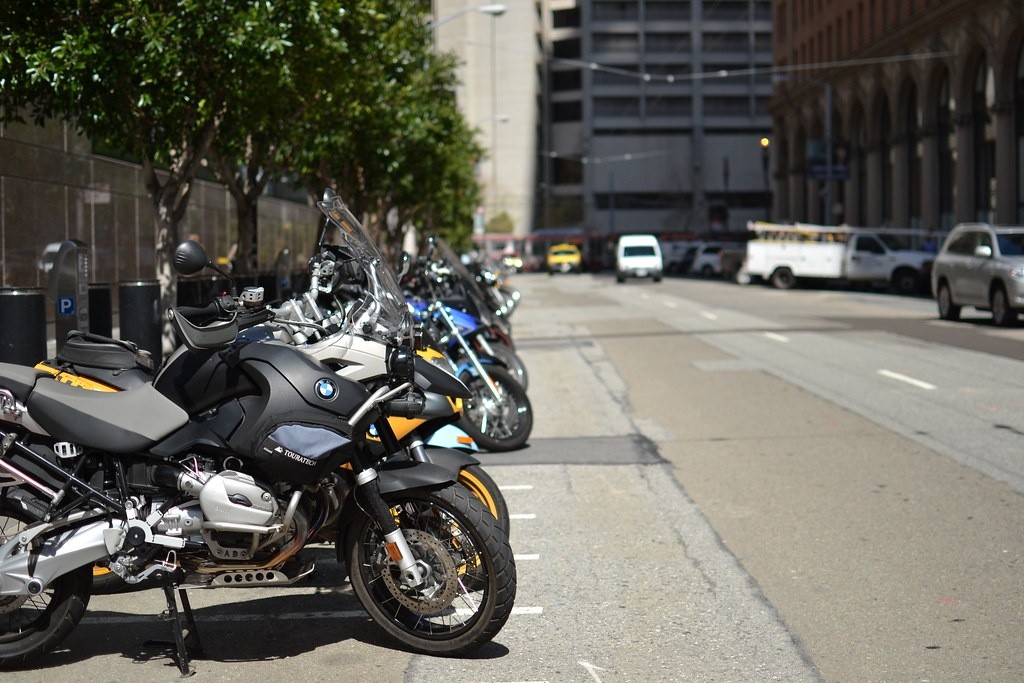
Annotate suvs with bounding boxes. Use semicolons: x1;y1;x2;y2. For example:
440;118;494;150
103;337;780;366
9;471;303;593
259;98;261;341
934;222;1024;329
659;238;746;283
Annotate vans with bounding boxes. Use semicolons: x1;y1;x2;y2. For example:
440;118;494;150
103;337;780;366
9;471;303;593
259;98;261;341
615;234;663;283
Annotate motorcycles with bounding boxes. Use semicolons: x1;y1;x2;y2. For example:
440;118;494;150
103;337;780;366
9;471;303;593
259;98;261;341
0;188;535;678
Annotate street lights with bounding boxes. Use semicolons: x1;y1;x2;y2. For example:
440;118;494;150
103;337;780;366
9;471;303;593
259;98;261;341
771;72;835;227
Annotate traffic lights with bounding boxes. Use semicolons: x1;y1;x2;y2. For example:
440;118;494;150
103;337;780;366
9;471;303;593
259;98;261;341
761;136;770;148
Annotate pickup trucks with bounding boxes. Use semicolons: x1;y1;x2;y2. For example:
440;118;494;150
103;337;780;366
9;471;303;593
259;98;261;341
743;225;938;296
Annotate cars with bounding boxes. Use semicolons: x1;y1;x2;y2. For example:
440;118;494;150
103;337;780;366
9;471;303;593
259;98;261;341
547;244;583;275
